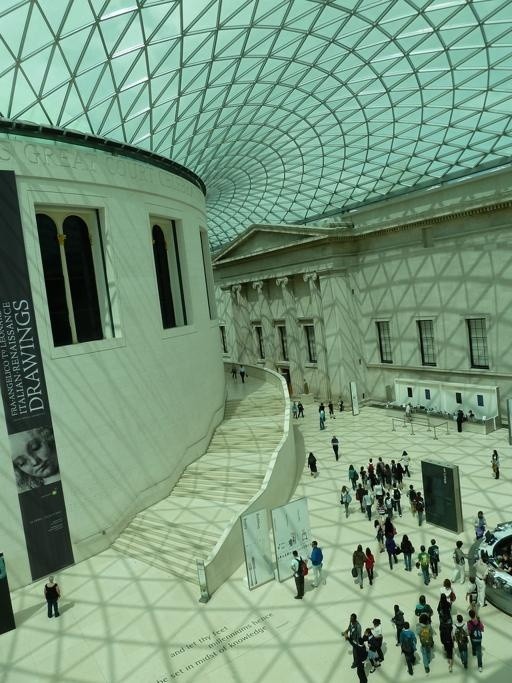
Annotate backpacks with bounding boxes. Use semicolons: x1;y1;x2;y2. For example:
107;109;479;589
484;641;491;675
295;557;308;577
417;625;434;649
453;624;469;647
470;622;482;642
402;634;415;654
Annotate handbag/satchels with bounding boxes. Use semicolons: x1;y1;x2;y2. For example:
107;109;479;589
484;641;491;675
449;592;457;601
352;568;358;577
396;547;400;555
470;592;477;605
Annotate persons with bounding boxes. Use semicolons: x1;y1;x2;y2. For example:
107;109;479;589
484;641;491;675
230;363;237;379
239;365;245;384
44;576;61;618
